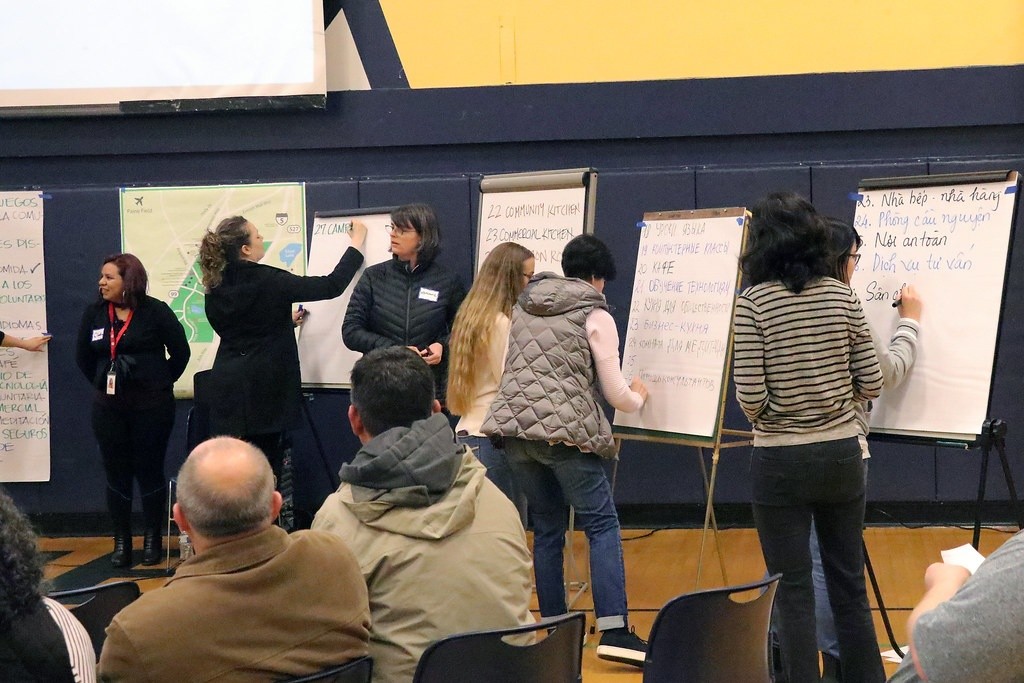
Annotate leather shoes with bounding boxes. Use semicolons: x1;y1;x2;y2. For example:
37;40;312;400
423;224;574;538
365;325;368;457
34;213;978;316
141;529;163;566
111;536;133;566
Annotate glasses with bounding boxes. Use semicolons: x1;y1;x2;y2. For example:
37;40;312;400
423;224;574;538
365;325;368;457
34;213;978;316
849;253;861;265
384;225;416;237
523;273;535;279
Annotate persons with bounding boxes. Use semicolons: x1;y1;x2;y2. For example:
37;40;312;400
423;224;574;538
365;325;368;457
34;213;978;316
310;346;537;683
199;216;368;493
481;234;648;667
731;191;889;683
0;494;96;683
762;217;923;683
103;437;372;683
443;243;535;502
341;203;467;417
887;529;1024;683
0;331;52;352
76;254;192;567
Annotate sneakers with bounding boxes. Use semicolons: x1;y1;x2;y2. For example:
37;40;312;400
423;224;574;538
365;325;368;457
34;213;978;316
597;625;648;668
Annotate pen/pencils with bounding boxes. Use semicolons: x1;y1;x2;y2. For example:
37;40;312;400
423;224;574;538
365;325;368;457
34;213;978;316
425;345;433;355
936;441;968;449
892;297;902;307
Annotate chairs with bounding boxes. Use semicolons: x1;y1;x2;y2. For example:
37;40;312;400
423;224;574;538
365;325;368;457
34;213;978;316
46;581;142;664
166;407;196;568
277;652;374;683
413;612;587;683
643;574;784;683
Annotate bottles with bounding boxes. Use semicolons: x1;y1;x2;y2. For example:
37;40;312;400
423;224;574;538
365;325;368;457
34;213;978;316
178;530;194;562
